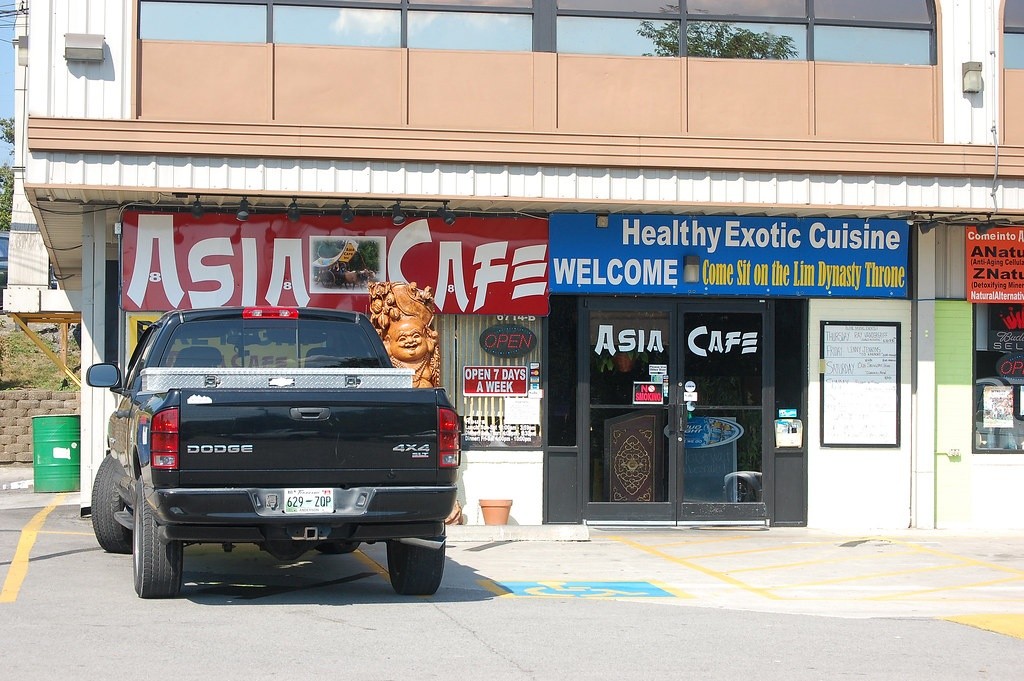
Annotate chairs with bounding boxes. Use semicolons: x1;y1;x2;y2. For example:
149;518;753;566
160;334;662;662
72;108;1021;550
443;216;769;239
303;347;347;367
172;345;224;367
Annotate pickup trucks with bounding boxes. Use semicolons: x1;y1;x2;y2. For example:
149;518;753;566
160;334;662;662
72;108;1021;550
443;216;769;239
86;306;463;599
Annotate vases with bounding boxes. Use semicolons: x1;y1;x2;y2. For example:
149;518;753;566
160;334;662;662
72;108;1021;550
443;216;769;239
479;499;513;525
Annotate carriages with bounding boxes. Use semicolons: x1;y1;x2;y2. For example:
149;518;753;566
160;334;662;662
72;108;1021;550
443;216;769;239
312;257;375;290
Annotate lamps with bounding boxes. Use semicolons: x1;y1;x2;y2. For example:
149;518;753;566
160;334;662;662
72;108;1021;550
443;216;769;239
962;62;982;93
595;214;609;229
436;202;457;226
237;195;249;222
918;214;940;235
392;202;406;226
341;199;354;223
62;32;106;64
975;215;997;235
286;198;300;221
191;192;203;217
